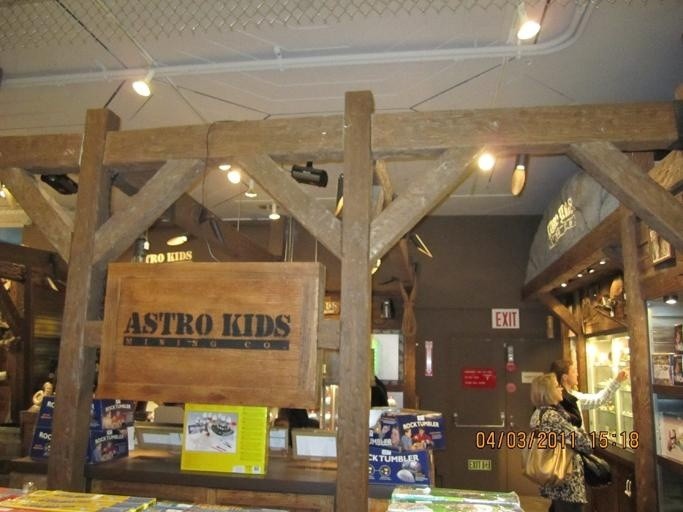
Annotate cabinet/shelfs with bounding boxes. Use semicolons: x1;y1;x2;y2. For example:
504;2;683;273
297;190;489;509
569;326;683;473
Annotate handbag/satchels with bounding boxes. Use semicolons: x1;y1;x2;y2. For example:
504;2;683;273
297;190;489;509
582;452;612;487
521;436;577;489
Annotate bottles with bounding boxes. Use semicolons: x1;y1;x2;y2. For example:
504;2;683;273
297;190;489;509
383;301;391;320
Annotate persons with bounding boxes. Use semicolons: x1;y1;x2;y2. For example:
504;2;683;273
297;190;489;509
522;372;594;512
94;441;117;461
390;426;432;449
103;410;124;426
550;359;630;434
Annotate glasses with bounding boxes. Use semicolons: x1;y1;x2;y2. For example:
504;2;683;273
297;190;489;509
555;383;563;390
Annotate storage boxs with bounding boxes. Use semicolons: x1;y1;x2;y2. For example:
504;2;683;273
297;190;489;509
28;396;135;465
363;407;447;487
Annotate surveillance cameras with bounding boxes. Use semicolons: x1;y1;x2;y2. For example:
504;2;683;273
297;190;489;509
292;165;328;186
41;173;78;194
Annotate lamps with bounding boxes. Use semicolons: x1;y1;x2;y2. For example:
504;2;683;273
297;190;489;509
288;156;330;189
